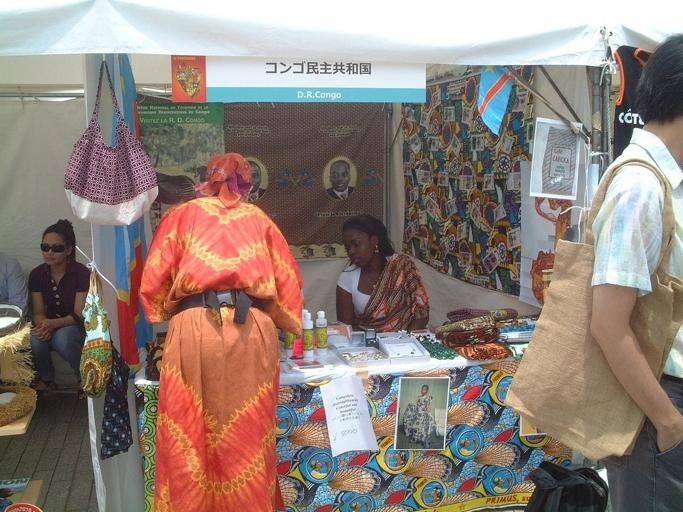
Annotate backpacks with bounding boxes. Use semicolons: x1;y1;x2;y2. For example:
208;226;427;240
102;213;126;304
525;464;608;512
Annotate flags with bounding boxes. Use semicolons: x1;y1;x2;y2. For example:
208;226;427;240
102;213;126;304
476;64;517;140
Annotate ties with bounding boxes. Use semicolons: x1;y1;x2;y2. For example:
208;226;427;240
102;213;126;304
251;195;257;200
340;193;346;201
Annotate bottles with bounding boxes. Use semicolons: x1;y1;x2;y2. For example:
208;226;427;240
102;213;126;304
284;308;328;358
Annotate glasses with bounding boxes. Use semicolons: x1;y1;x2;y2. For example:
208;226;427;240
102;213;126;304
41;244;69;253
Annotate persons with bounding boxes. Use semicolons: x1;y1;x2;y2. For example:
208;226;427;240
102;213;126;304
403;382;439;450
0;486;15;511
0;252;30;324
243;160;266;199
327;160;354;197
29;218;90;400
588;31;683;512
336;211;431;335
137;151;304;512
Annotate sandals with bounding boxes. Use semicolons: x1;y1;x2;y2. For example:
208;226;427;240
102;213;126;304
78;380;87;400
34;378;53;395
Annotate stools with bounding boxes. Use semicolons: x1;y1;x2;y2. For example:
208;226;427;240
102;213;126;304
46;349;80;394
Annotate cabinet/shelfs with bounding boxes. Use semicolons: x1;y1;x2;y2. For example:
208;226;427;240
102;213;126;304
0;324;37;436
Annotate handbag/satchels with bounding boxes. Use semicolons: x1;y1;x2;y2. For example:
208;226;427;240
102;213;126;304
64;61;159;225
79;293;113;398
506;239;683;462
101;348;132;459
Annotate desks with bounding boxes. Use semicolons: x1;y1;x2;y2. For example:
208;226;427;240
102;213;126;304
134;330;605;512
